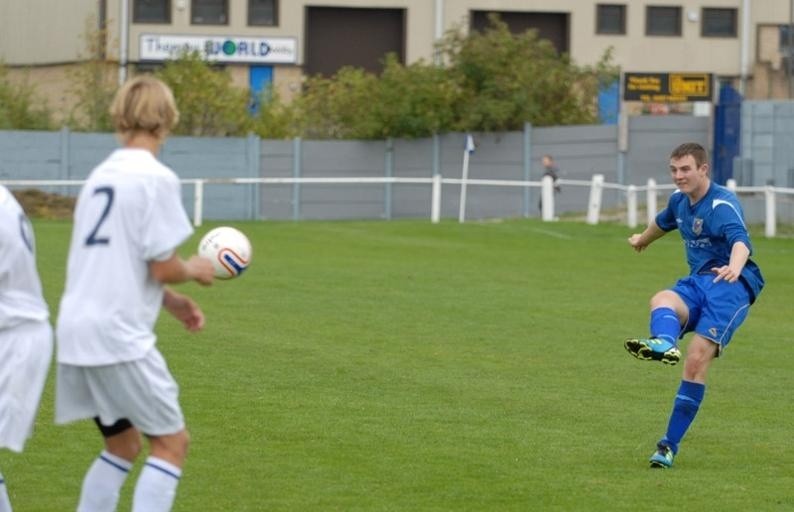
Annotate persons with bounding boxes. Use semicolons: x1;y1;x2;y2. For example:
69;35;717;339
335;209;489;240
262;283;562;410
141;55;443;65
538;155;561;215
52;71;215;510
622;141;765;469
0;179;54;511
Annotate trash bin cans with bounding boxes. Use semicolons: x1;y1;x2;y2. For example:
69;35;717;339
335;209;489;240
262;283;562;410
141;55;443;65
732;156;753;187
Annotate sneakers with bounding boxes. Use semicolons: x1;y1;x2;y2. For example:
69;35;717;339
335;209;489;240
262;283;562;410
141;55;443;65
650;441;675;468
625;338;681;366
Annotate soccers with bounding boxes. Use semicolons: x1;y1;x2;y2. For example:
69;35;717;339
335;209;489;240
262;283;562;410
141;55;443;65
198;226;252;279
